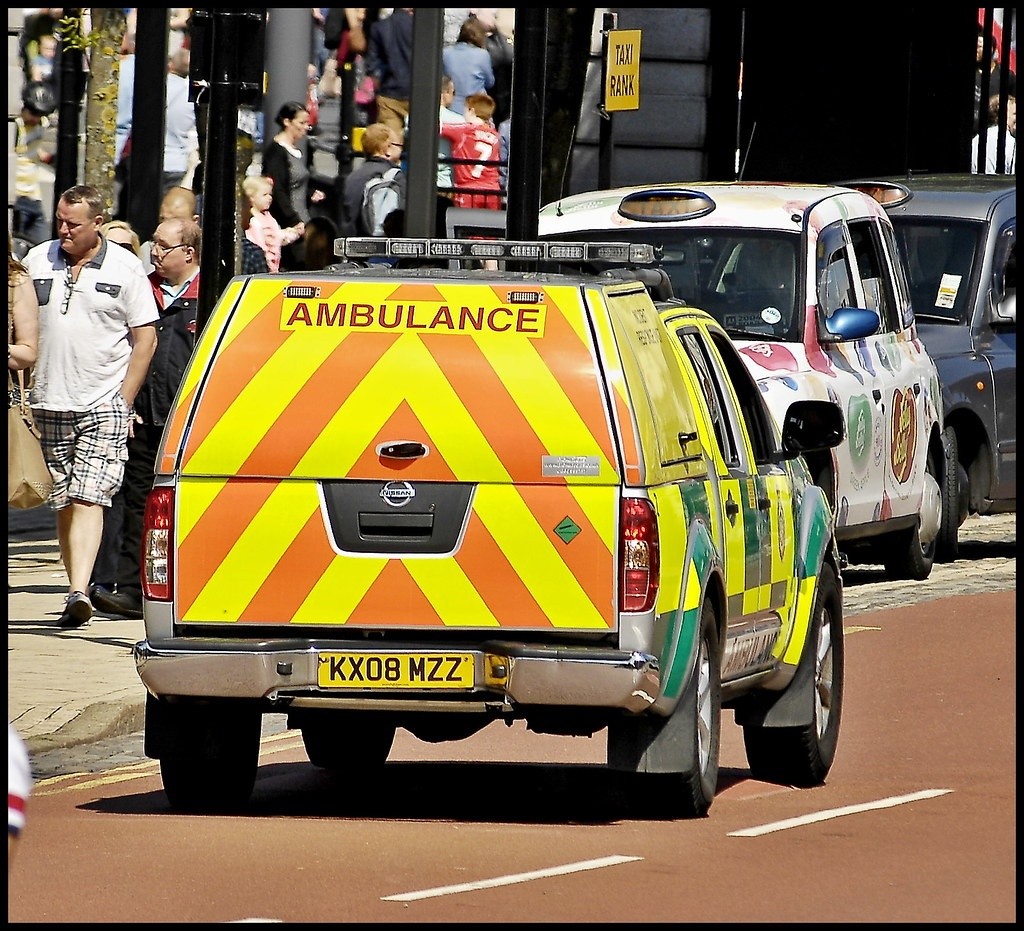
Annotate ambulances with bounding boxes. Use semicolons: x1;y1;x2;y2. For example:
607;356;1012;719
131;240;853;824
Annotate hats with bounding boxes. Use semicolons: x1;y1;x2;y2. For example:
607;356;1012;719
105;228;138;256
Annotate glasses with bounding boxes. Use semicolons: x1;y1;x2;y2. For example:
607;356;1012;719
150;240;192;257
447;88;456;97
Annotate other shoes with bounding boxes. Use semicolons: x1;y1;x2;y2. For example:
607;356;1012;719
90;586;143;619
57;592;91;629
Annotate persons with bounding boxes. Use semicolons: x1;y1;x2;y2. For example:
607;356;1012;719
16;8;63;163
20;183;161;628
306;6;515;270
8;228;40;369
116;7;197;199
721;240;791;314
971;30;1016;175
789;409;838;450
88;186;201;622
242;100;342;276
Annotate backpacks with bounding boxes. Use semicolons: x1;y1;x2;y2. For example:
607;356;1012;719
361;168;407;237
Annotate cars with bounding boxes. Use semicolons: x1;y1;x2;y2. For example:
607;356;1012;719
701;170;1015;565
532;180;951;582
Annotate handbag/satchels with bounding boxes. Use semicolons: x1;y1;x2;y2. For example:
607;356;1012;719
8;368;52;509
